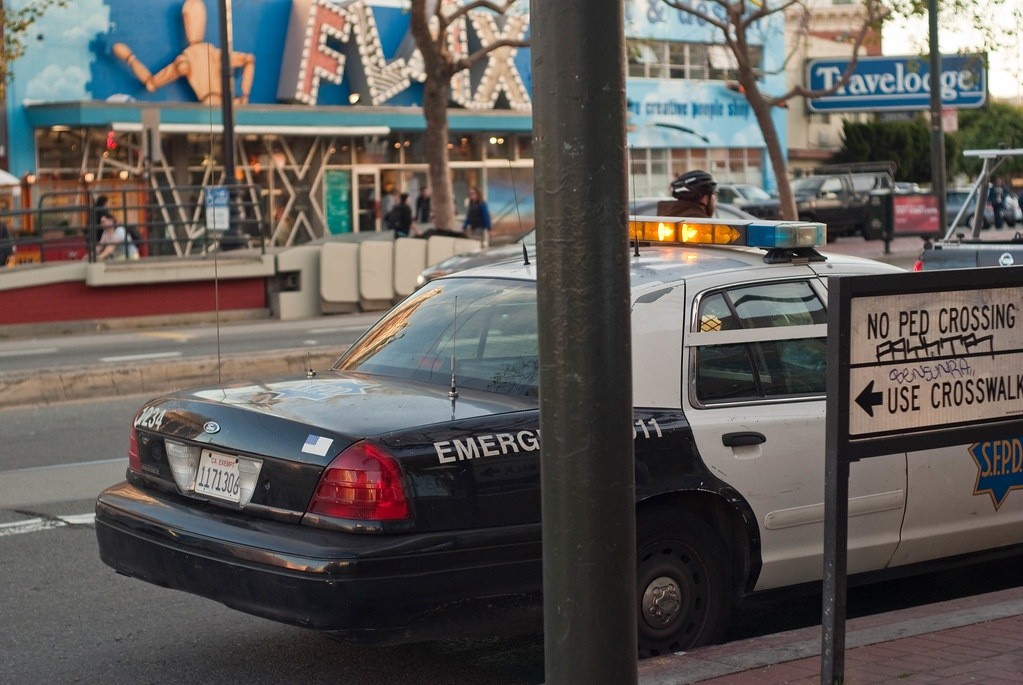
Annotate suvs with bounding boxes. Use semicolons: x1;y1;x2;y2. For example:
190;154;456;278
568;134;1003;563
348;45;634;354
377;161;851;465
740;159;901;245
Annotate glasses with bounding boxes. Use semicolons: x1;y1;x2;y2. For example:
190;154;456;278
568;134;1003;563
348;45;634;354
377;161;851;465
709;189;719;197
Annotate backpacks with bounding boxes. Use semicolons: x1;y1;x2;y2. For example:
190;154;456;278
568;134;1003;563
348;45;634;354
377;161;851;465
386;206;402;229
114;223;142;248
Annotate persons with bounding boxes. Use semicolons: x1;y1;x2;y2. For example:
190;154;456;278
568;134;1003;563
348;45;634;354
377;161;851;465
83;214;140;260
987;177;1009;230
463;187;492;247
93;196;116;241
381;184;430;237
0;223;18;267
657;169;719;219
274;207;301;247
114;0;255;104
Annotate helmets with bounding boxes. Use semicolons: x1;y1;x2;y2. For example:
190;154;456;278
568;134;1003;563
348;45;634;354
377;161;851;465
671;169;717;200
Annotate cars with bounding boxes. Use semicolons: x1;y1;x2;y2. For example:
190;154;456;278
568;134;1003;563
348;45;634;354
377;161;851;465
716;183;772;203
94;213;1023;661
629;196;762;220
947;185;1003;230
